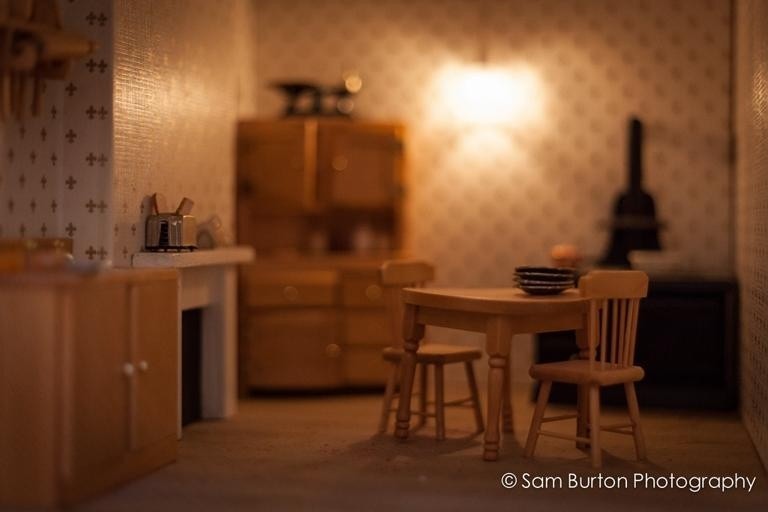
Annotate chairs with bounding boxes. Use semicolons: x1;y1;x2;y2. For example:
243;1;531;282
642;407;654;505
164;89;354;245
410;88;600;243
526;267;650;464
377;260;485;443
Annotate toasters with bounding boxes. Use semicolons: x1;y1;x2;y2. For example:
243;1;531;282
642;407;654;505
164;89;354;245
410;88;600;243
142;191;202;254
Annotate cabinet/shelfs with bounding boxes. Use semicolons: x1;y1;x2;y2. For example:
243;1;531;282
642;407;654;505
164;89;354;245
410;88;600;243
0;244;180;512
531;263;742;415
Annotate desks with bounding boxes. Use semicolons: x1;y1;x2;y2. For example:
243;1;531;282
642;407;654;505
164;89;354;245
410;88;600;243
393;284;601;462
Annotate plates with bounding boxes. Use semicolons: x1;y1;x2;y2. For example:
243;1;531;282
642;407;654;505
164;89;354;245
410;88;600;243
512;266;574;297
